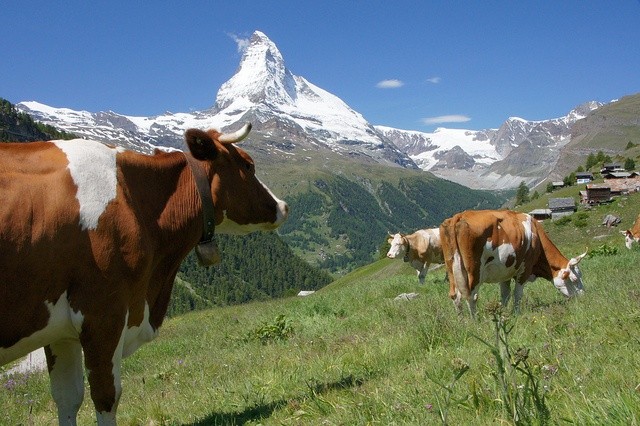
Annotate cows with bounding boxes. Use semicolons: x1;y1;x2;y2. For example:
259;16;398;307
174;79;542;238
440;209;589;319
625;213;640;249
0;122;289;426
387;227;449;282
